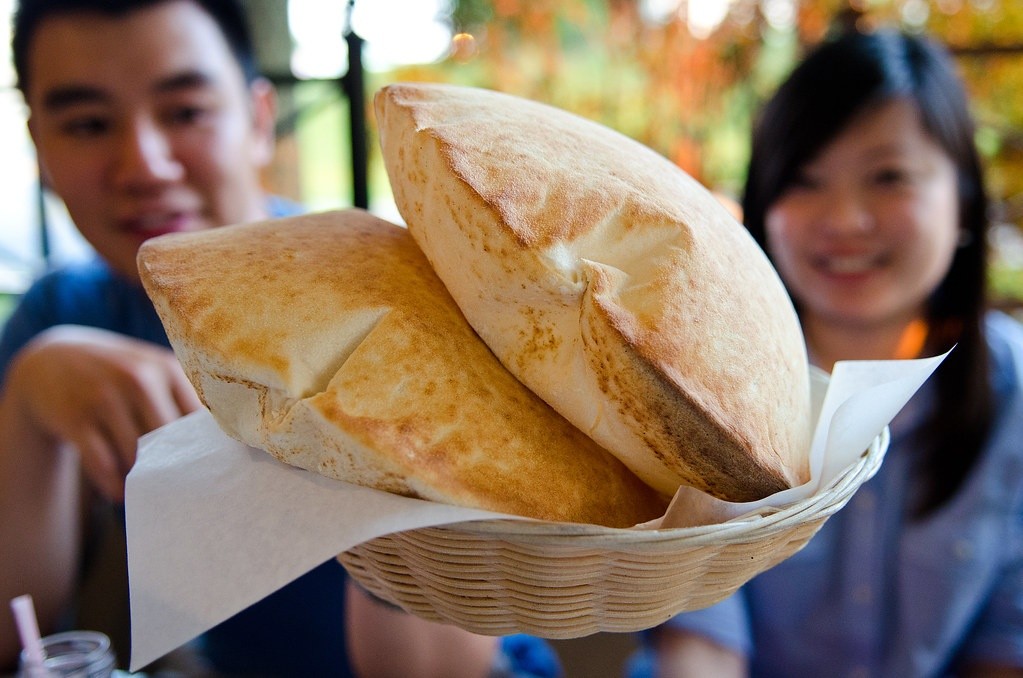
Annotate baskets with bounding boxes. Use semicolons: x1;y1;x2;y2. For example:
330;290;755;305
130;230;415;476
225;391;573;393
336;368;890;639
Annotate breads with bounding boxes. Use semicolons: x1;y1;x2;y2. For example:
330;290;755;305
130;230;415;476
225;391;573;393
374;83;810;503
135;210;677;528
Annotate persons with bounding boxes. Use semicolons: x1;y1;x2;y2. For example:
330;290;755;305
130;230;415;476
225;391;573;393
0;0;499;678
652;32;1023;678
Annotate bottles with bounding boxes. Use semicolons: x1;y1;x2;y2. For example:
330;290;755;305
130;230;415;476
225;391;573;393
18;630;146;678
